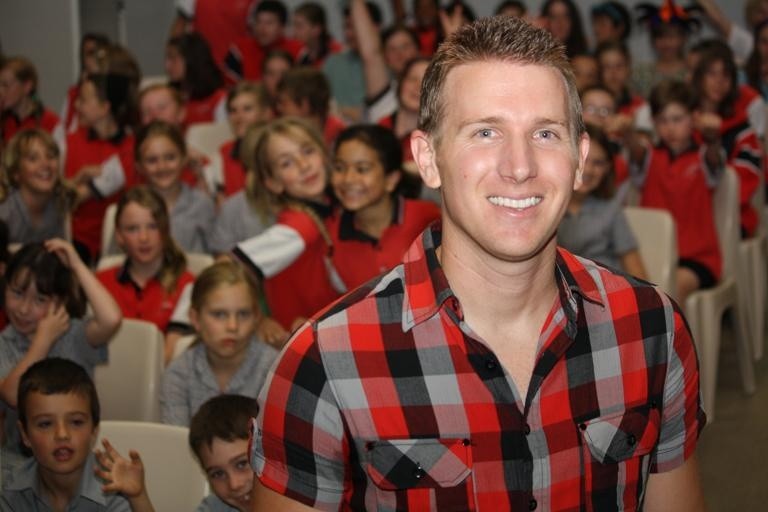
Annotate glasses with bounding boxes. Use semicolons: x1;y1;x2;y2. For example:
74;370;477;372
580;104;614;119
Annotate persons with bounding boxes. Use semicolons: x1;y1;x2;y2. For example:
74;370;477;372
0;357;133;512
157;260;283;429
1;0;767;371
247;15;706;510
92;393;259;512
0;238;122;483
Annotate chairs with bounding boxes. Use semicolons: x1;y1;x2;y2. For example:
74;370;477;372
625;206;680;303
94;320;161;420
186;120;234;187
738;174;767;360
85;420;206;511
84;250;213;316
682;166;755;423
